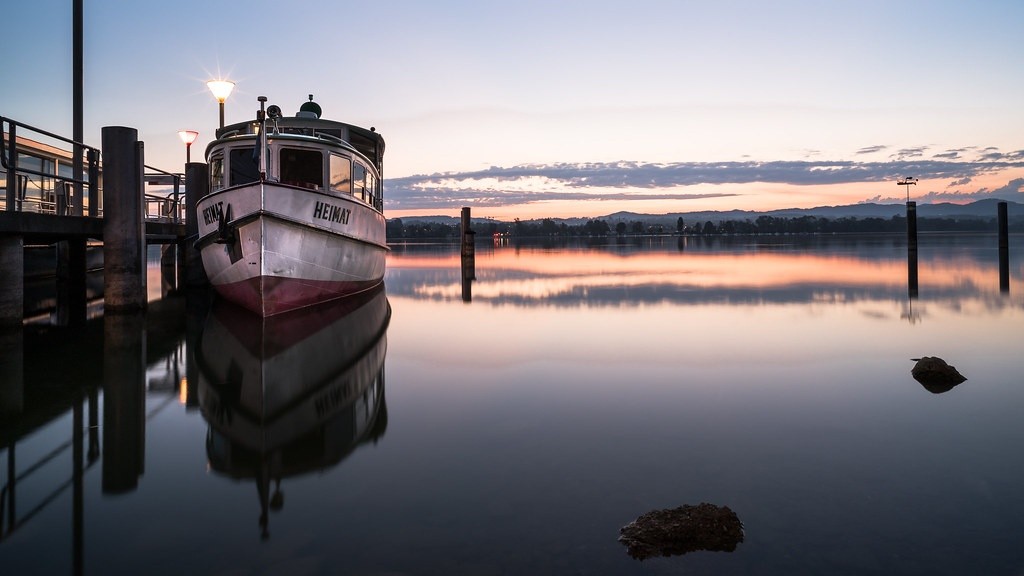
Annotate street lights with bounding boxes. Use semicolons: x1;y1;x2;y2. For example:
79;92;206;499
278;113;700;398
207;79;235;129
178;130;199;164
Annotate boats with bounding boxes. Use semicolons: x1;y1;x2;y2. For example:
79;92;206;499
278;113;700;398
197;281;393;535
197;94;388;320
